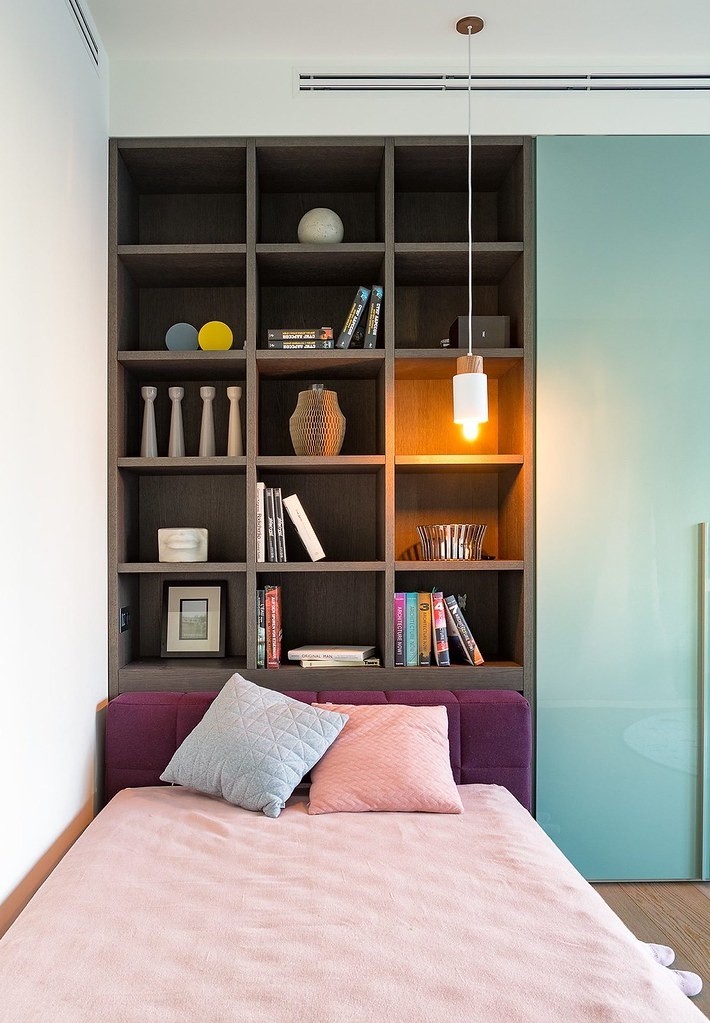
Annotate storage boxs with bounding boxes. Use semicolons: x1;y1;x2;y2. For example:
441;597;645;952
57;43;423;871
450;316;511;348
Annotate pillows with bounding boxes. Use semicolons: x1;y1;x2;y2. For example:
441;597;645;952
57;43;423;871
308;702;464;816
159;673;349;817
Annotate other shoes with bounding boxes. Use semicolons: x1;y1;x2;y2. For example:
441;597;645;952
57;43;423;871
647;943;675;967
670;969;703;996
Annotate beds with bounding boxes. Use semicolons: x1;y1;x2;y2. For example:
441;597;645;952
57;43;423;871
1;689;710;1023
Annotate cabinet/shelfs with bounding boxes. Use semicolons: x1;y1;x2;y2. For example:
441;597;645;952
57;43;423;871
536;134;710;884
107;135;536;820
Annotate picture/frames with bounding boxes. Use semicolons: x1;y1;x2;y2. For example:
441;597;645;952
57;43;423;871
161;580;228;658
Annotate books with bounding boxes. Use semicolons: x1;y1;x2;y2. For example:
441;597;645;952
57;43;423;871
257;585;282;669
289;645;381;668
257;482;326;563
268;285;382;350
394;592;484;668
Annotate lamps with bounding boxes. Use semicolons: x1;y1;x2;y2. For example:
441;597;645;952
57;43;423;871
453;15;489;442
